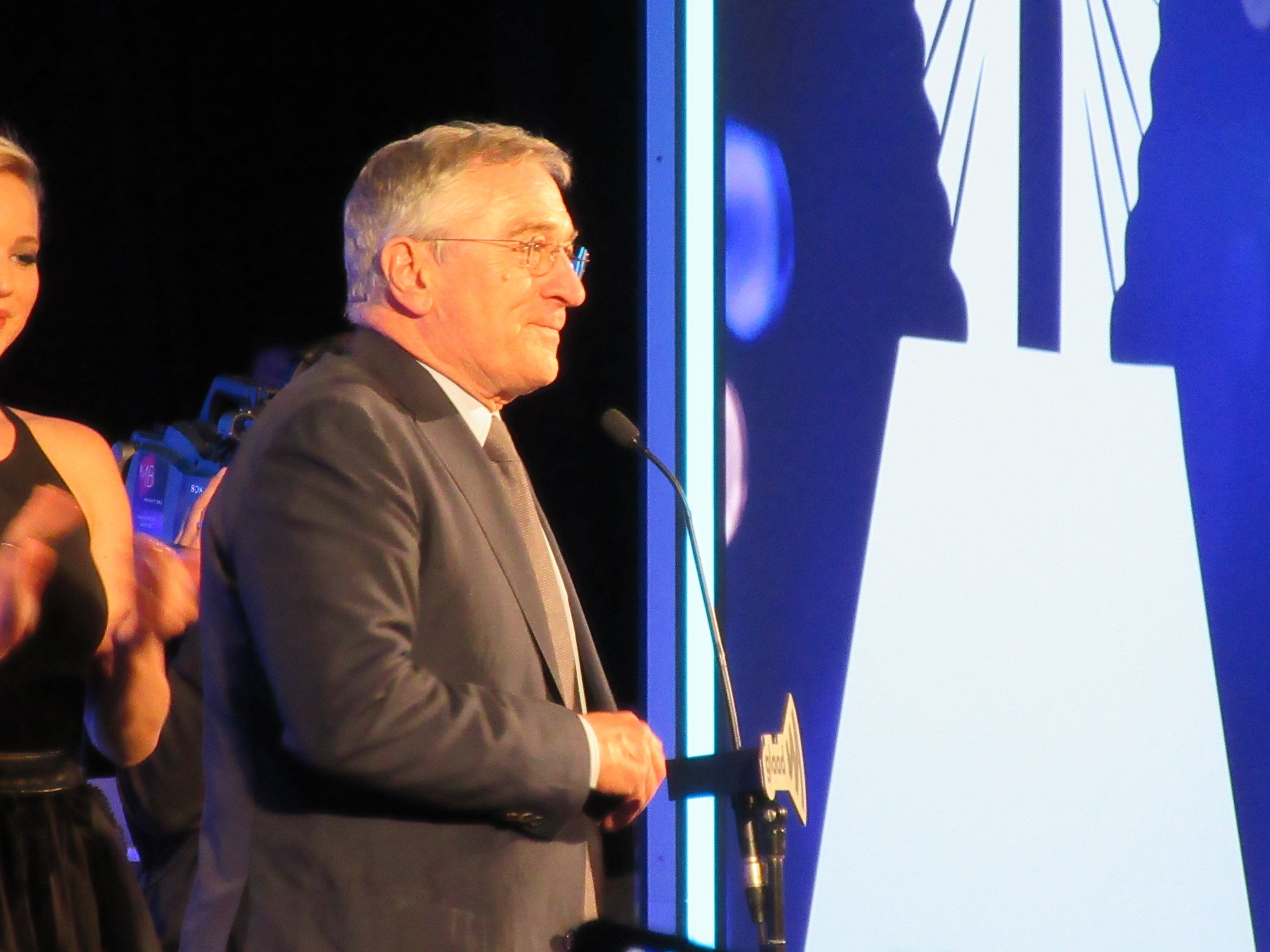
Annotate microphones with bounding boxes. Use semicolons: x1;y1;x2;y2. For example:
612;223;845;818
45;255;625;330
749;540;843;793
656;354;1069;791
602;405;787;952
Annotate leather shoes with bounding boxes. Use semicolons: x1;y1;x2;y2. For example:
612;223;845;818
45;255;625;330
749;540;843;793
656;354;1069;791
483;415;585;714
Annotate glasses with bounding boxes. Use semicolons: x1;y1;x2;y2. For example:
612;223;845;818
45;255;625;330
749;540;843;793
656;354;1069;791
409;236;590;281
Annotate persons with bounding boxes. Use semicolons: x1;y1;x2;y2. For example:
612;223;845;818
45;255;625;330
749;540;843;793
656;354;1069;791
114;464;231;952
184;119;667;951
0;135;205;952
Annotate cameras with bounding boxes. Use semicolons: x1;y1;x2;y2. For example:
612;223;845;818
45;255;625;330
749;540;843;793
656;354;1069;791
107;378;275;545
